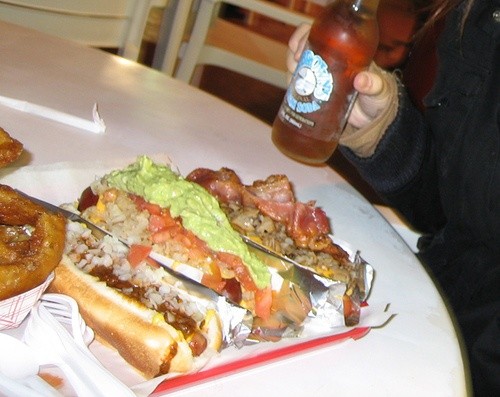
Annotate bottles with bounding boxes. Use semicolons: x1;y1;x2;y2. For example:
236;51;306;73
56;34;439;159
271;0;381;167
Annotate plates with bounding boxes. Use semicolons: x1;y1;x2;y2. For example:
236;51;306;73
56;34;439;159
0;19;471;397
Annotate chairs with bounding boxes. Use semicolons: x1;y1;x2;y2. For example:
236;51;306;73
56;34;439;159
176;0;315;119
0;0;154;62
152;0;268;107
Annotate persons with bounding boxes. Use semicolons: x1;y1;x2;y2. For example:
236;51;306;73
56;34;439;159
285;1;500;397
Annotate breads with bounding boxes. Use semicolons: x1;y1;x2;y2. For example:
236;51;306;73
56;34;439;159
0;183;65;303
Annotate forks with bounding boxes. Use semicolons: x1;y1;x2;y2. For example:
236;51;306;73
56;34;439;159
24;294;137;397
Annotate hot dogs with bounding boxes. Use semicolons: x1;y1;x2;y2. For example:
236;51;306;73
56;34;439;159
56;223;223;377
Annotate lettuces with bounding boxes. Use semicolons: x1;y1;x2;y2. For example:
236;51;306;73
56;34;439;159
105;156;270;287
